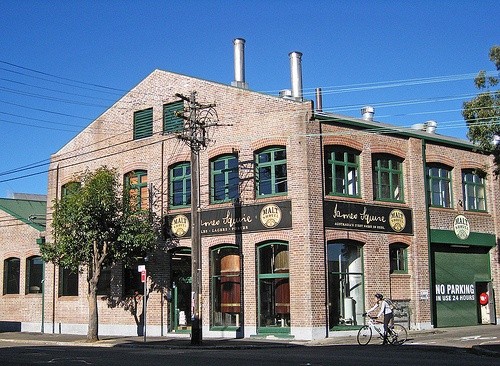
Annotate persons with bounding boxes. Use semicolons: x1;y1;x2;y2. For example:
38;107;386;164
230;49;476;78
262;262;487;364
362;294;398;346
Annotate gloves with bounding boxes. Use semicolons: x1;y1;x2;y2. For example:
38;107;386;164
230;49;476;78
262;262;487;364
362;312;367;317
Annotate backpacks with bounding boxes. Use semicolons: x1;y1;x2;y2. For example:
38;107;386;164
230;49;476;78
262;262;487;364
378;299;396;312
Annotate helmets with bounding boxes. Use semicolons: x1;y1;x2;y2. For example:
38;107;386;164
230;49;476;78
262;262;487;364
375;294;383;298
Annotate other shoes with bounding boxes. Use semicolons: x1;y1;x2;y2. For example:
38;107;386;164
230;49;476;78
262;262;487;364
381;342;388;346
391;334;398;340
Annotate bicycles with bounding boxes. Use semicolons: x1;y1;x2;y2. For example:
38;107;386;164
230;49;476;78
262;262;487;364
357;313;408;346
